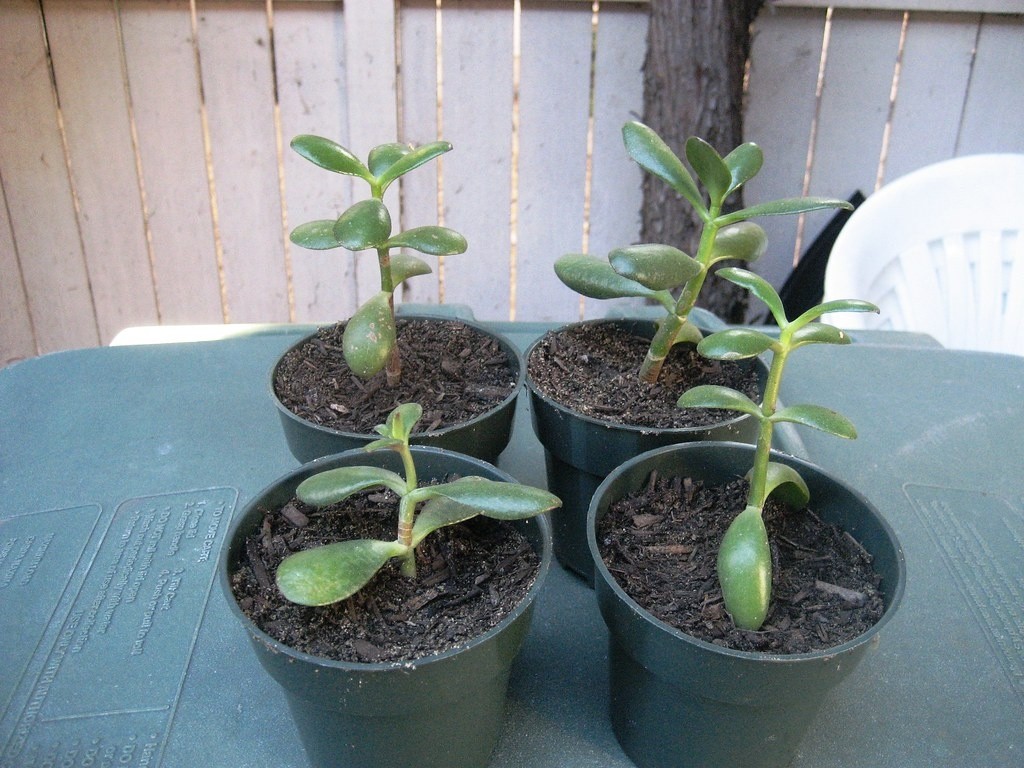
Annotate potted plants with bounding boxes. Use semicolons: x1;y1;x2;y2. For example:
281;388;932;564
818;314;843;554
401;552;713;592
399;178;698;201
586;267;905;768
270;134;524;470
523;122;853;590
221;403;560;768
